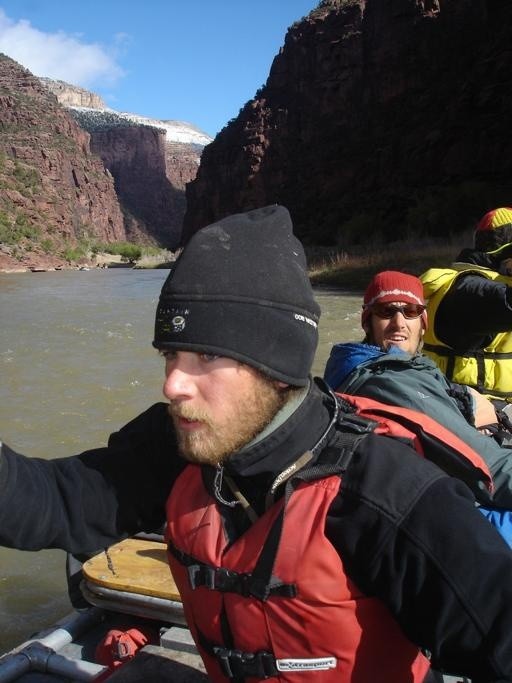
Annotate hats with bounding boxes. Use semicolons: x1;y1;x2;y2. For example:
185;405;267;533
151;201;324;391
359;268;430;332
472;206;512;254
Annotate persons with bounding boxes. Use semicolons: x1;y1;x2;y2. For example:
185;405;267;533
1;203;510;681
323;270;511;542
418;204;510;404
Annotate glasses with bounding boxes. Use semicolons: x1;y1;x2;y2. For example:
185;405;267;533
361;299;428;320
